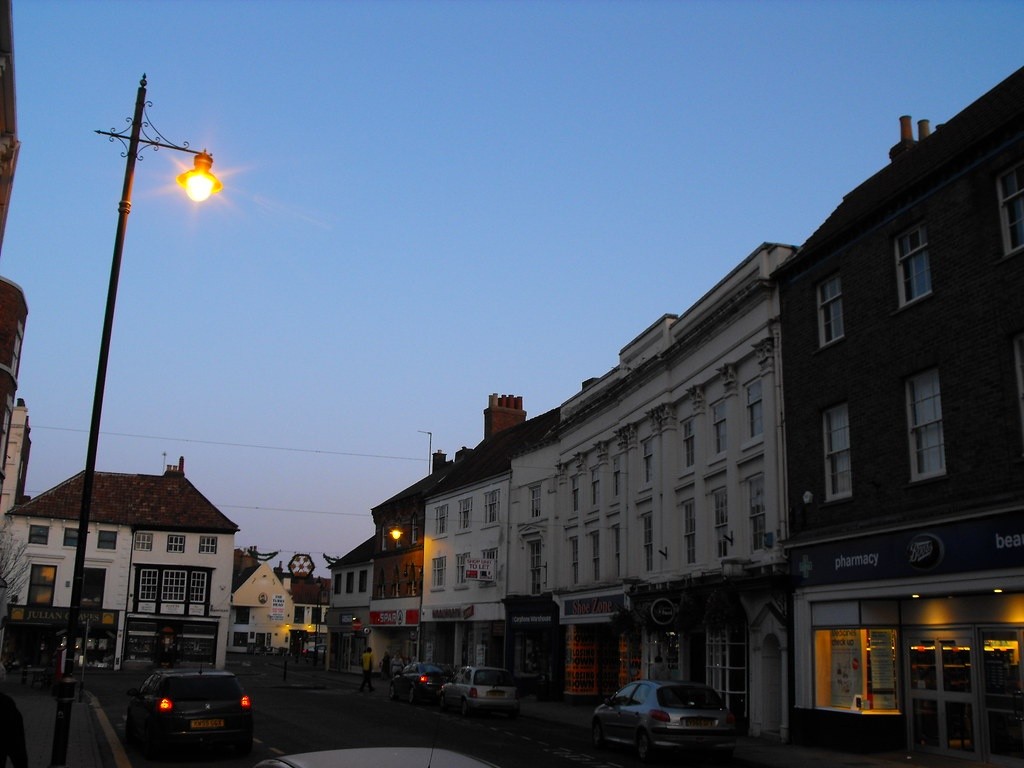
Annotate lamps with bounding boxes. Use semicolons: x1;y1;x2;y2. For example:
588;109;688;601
388;520;421;540
351;615;360;623
403;563;422;578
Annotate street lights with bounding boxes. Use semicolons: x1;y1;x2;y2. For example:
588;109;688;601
48;70;224;767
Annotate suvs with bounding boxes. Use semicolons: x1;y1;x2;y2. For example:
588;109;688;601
438;665;519;718
589;678;739;759
122;669;255;757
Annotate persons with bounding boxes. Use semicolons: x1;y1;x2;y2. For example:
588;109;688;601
0;661;28;768
651;656;671;682
359;647;377;693
379;650;405;681
294;648;319;666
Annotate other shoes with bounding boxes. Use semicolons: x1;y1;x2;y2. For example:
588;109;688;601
359;688;363;691
369;688;374;692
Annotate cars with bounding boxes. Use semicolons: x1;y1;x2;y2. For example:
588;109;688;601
388;661;448;704
253;745;503;768
